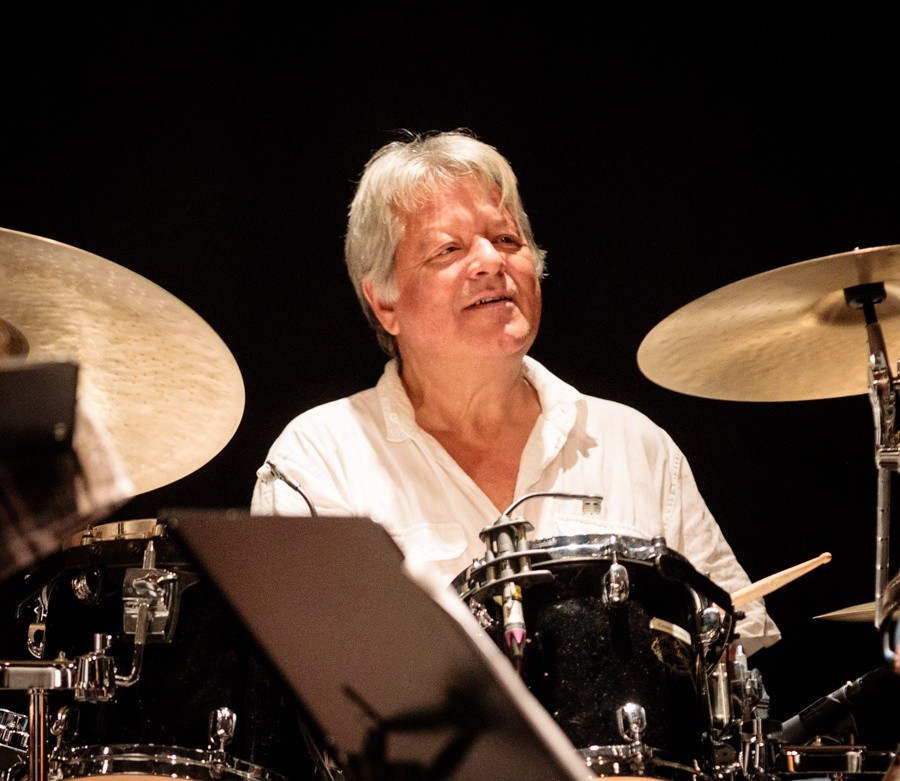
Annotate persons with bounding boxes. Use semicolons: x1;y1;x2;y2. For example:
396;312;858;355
251;128;778;685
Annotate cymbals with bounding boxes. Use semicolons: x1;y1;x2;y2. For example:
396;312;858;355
636;244;900;402
0;227;245;512
812;600;900;622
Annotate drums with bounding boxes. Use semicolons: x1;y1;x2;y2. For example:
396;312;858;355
0;517;335;780
452;537;719;781
